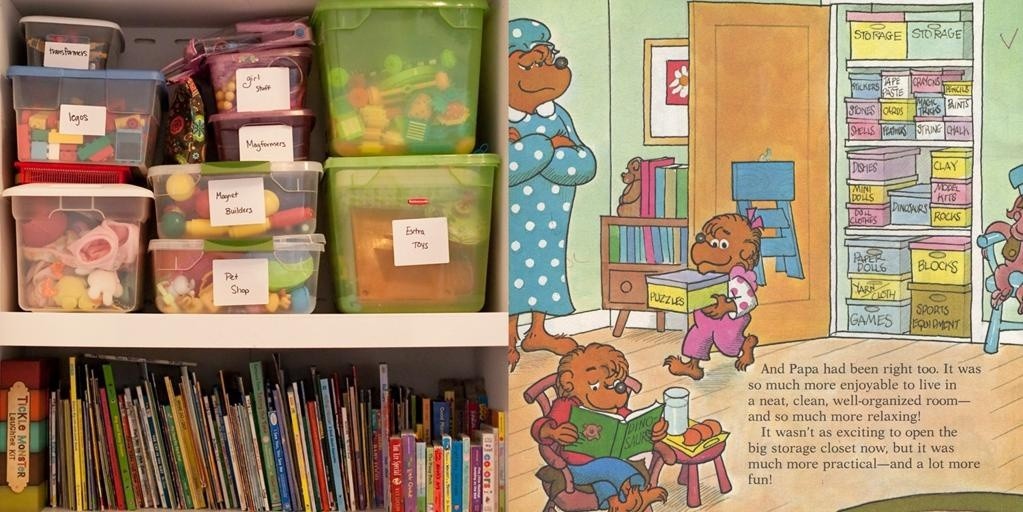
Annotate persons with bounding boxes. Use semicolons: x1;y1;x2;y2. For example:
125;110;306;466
617;156;642;215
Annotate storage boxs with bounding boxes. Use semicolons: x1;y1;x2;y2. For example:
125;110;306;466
0;0;505;511
146;158;326;236
312;0;496;158
147;232;324;313
17;15;128;68
202;46;311;113
9;63;168;180
1;181;156;310
208;110;316;163
325;156;499;316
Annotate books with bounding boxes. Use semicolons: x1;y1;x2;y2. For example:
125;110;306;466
642;153;688;218
609;223;688;266
562;400;666;460
48;350;508;512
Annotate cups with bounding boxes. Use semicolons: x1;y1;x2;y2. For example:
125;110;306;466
662;386;690;436
